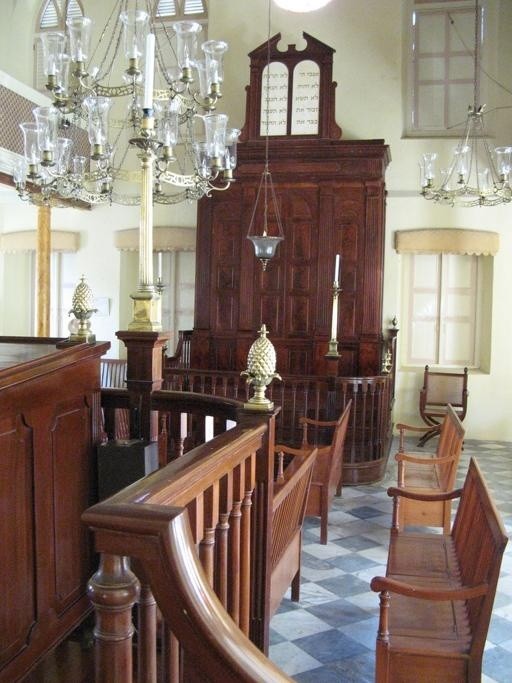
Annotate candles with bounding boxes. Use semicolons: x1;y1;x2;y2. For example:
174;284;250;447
335;255;341;285
157;252;162;279
144;33;155;107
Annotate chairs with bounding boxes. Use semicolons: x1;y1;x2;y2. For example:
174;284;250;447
418;364;469;451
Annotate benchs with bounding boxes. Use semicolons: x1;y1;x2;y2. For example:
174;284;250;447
370;457;510;683
395;402;465;534
275;394;353;544
270;442;319;620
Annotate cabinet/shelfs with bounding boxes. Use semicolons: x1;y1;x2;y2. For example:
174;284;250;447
0;338;112;682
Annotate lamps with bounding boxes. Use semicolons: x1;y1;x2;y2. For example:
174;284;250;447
418;1;512;206
11;0;242;207
245;0;285;272
